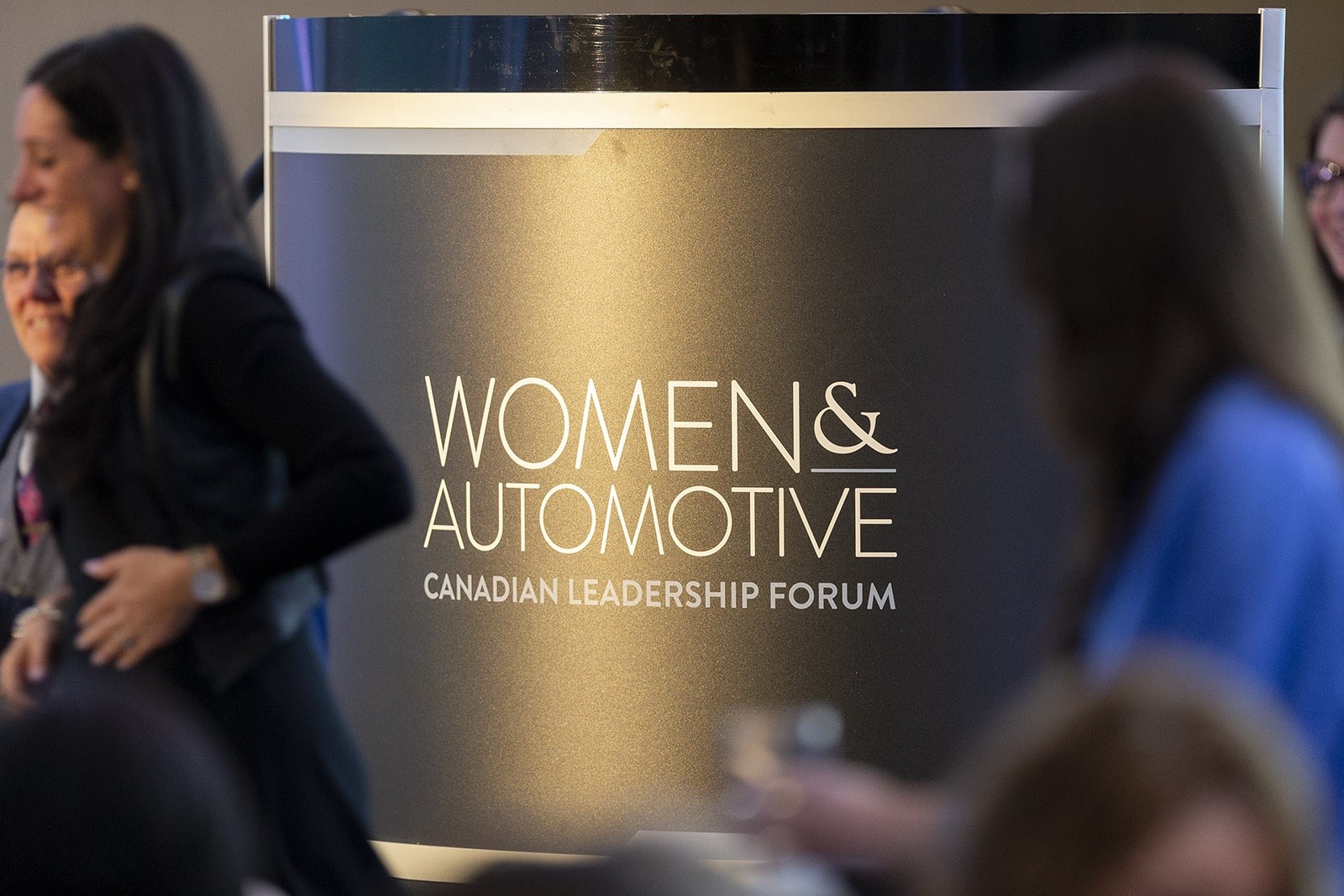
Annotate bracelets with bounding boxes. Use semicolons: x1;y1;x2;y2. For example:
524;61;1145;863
15;603;69;620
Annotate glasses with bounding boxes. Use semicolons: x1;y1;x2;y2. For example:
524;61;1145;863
1292;159;1344;202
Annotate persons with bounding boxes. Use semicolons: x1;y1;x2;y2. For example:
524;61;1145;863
942;653;1314;896
731;54;1344;896
0;22;415;896
0;200;328;676
1302;90;1344;304
0;675;286;896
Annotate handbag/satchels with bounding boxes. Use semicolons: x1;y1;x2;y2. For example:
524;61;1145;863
187;563;325;688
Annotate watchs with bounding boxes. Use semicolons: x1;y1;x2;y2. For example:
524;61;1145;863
177;541;229;605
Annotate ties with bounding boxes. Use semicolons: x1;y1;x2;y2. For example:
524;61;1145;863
16;396;52;548
